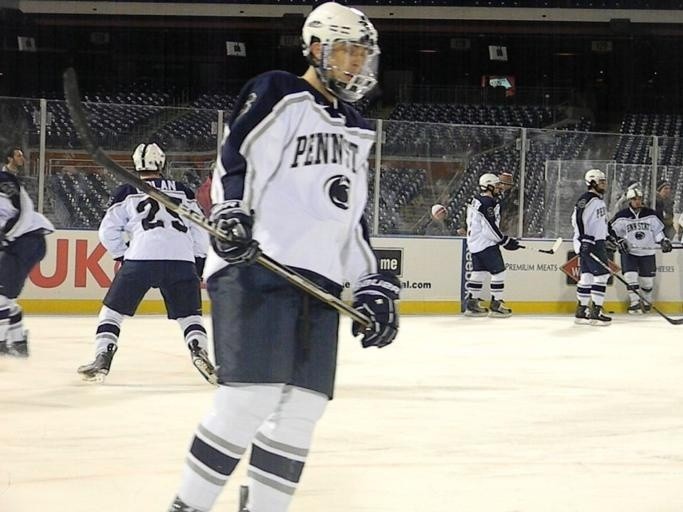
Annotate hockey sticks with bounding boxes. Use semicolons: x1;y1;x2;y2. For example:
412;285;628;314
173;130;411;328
590;252;683;324
519;237;562;254
63;67;374;329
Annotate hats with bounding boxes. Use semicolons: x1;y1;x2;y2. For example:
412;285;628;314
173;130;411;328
657;179;671;191
432;204;446;217
498;172;513;186
626;180;642;189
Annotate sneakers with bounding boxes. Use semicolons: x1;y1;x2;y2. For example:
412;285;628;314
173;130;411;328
77;353;112;375
0;338;28;358
490;300;511;314
627;303;651;311
466;299;488;313
191;347;218;385
590;305;612;321
576;306;591;319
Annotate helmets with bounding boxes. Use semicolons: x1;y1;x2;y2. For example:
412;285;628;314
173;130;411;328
478;173;500;191
302;2;381;103
627;188;642;199
585;169;606;187
132;142;166;171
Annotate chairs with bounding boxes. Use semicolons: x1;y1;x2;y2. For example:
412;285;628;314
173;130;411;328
14;81;683;246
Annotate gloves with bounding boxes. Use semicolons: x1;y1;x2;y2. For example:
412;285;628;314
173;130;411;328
616;238;630;255
352;271;401;347
580;235;595;256
661;238;672;253
207;199;261;267
604;236;618;252
499;236;519;250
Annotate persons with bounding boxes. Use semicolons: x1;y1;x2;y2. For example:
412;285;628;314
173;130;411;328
78;142;217;386
2;148;24;176
0;171;54;358
614;181;642;213
425;205;466;236
571;169;612;326
656;180;671;239
607;188;672;314
167;2;399;512
464;174;519;317
